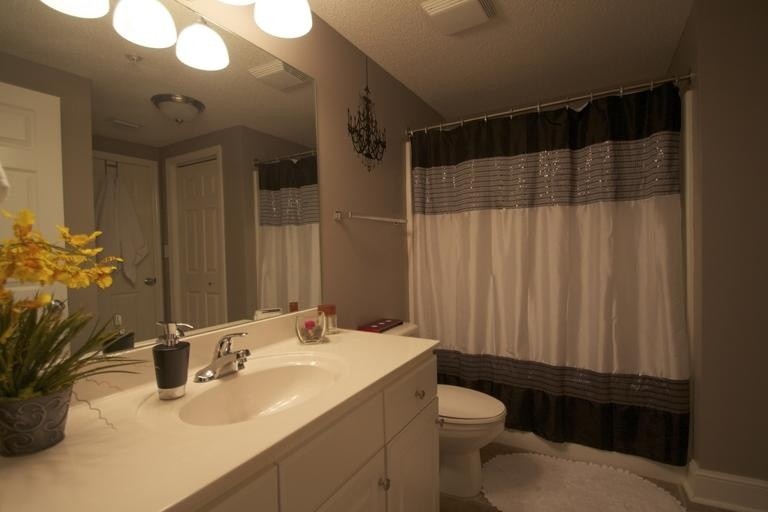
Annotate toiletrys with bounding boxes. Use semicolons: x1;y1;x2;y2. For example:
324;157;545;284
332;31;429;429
150;319;194;401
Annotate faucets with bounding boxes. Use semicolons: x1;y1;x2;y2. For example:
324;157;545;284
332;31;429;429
192;332;252;383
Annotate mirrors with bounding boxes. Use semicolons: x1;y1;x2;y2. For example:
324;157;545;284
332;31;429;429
3;1;325;372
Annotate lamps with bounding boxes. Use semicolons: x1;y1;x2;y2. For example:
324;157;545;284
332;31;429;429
346;56;387;174
150;93;204;123
42;0;109;18
177;18;229;71
253;0;313;38
247;59;311;92
421;0;494;36
113;0;175;48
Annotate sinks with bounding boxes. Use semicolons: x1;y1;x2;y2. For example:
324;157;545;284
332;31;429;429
171;354;346;427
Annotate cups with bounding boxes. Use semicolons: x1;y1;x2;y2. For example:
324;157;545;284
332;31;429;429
296;313;326;345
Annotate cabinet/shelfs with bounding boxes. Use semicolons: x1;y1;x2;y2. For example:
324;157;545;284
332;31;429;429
164;444;277;512
277;341;439;512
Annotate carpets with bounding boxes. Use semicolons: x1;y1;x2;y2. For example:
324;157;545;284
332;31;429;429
482;453;686;512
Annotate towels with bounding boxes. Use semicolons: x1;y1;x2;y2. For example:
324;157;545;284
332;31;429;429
95;175;148;285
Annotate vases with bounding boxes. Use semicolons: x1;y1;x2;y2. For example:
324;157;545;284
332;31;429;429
0;383;73;458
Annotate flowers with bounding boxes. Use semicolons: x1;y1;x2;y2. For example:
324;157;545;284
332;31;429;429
0;205;150;382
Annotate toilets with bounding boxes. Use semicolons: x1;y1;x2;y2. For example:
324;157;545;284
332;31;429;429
381;320;506;498
